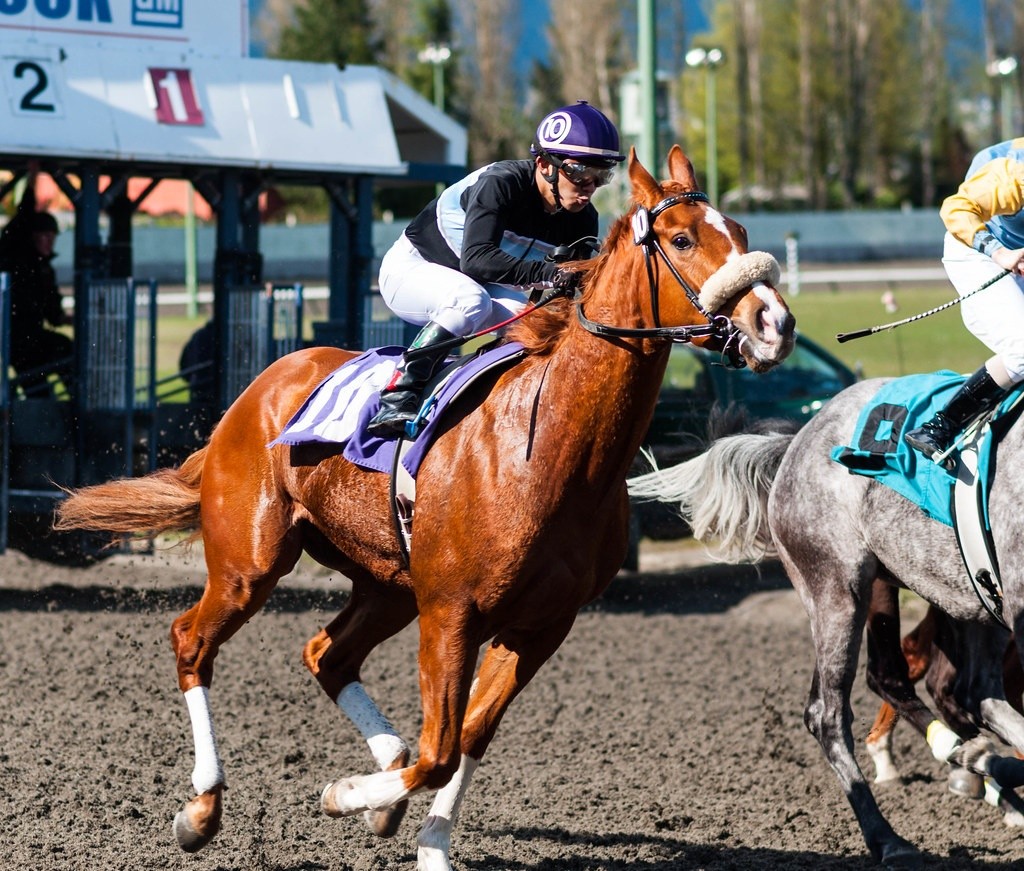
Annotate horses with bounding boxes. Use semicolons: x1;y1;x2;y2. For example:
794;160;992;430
43;141;799;871
624;365;1024;871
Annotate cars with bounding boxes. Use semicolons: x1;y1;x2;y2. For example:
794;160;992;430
636;329;863;513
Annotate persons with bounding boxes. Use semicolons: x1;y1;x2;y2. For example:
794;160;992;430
903;136;1024;471
366;99;627;441
179;319;218;445
0;211;78;400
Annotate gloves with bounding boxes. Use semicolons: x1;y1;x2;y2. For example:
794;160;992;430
553;260;581;288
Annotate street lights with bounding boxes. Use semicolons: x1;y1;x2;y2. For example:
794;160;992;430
416;42;451;113
986;56;1020;149
681;47;728;214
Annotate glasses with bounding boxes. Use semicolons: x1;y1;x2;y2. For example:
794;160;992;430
550;155;615;188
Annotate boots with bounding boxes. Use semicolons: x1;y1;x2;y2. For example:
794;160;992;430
367;320;457;438
905;366;1009;470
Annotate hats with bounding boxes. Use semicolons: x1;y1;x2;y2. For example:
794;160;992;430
530;100;626;162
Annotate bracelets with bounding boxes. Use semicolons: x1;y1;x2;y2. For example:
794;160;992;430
980;235;994;253
